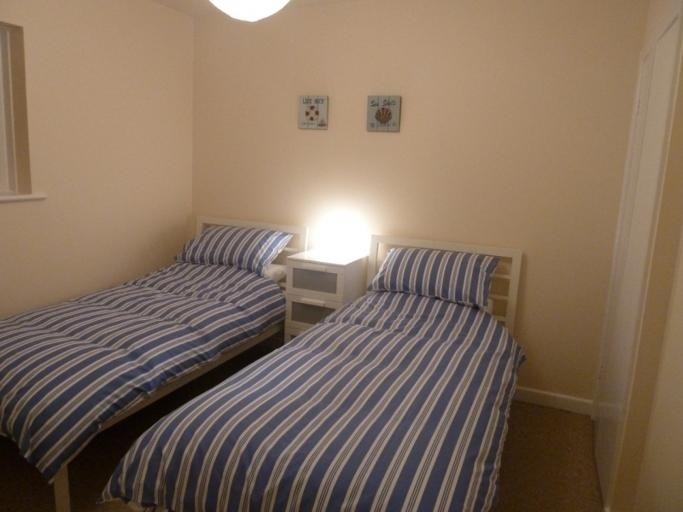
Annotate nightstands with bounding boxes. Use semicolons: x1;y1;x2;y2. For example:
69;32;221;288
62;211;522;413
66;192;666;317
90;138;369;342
284;243;369;344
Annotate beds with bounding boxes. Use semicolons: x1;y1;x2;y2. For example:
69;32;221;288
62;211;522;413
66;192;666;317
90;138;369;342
0;212;310;511
103;228;524;510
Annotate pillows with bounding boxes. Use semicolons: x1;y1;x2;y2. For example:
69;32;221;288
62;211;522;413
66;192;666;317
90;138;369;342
173;222;295;278
369;247;500;313
